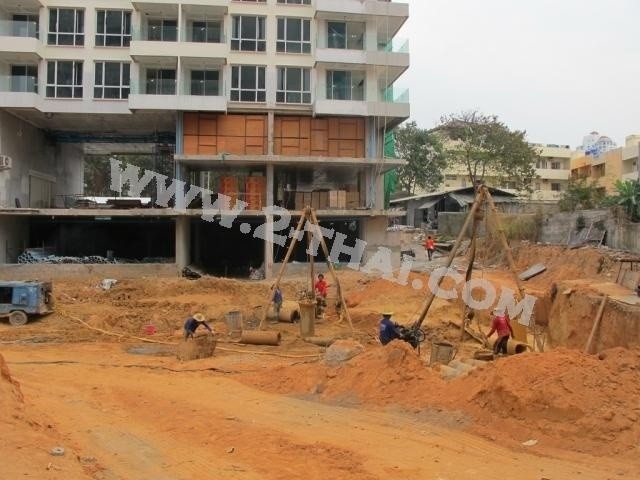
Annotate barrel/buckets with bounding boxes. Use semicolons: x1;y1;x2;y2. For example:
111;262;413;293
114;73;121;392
225;310;242;332
430;341;454;364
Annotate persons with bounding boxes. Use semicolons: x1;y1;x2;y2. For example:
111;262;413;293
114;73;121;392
271;284;282;324
315;274;327;315
184;313;214;342
485;310;515;354
426;236;434;261
379;312;400;345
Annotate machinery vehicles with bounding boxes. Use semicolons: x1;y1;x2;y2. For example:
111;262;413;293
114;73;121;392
0;277;55;328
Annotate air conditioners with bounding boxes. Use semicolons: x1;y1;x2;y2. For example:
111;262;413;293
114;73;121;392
0;152;14;170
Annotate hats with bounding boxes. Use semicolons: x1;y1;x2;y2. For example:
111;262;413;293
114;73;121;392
382;307;393;315
192;313;205;322
